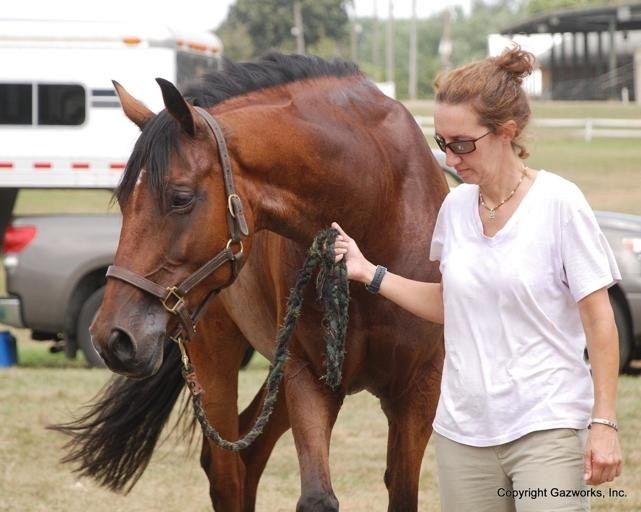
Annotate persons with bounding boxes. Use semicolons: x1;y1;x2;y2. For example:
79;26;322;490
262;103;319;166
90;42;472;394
321;44;624;512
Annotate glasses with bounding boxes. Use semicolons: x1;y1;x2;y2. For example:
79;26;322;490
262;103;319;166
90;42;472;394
435;129;495;157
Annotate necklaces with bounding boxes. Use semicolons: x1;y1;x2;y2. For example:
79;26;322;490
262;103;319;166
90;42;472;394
475;166;528;219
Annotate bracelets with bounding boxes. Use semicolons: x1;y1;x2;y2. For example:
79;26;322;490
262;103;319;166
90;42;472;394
586;416;620;433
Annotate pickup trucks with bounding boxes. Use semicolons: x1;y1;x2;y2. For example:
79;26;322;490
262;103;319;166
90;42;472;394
0;149;640;373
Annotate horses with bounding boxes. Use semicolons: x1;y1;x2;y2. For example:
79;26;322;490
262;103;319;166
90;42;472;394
48;49;454;512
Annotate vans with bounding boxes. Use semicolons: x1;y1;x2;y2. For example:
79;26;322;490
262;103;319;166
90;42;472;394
0;37;217;218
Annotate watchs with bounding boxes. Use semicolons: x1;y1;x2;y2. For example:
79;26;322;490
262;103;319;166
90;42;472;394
364;262;387;296
